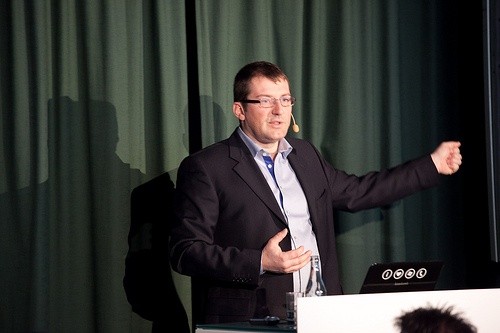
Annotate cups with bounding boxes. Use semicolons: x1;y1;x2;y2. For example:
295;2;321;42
286;292;304;325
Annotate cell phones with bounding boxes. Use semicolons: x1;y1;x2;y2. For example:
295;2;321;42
249;316;280;325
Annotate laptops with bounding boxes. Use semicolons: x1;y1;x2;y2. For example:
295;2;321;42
358;260;443;294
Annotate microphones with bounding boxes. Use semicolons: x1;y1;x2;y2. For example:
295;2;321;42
291;113;299;132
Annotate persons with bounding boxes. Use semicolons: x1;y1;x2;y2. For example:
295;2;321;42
169;61;462;333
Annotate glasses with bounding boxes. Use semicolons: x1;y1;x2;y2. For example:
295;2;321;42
241;96;296;108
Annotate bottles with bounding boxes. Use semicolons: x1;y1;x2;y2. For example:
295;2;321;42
304;255;327;296
